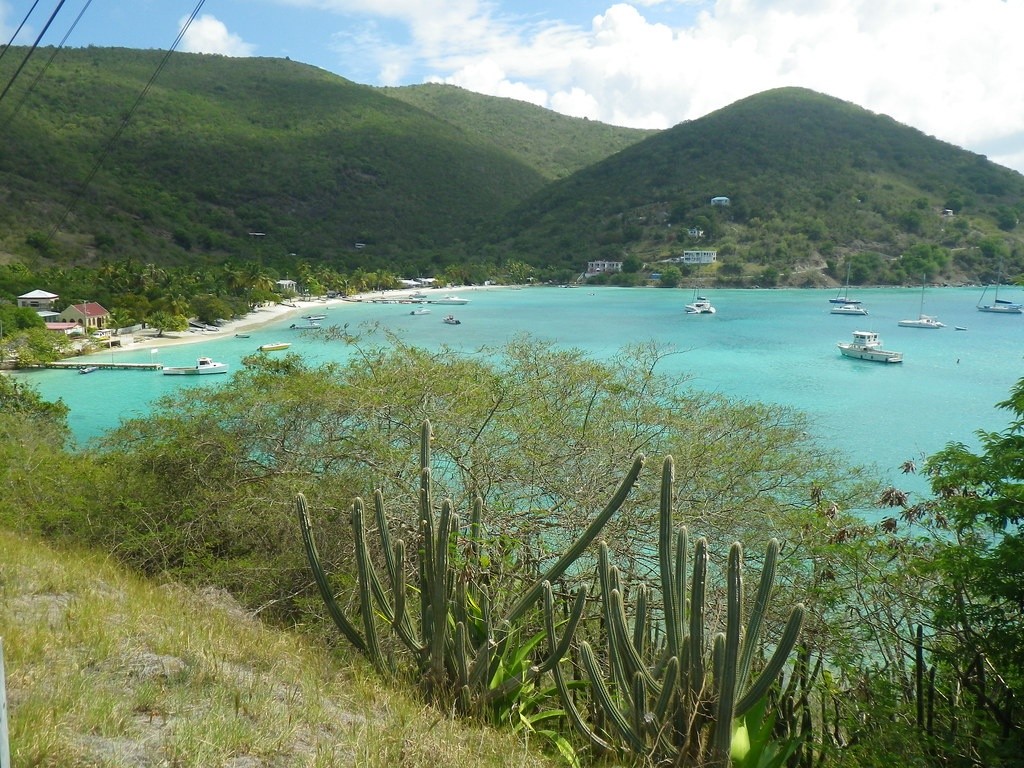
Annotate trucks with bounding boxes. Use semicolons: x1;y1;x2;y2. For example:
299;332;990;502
93;329;111;339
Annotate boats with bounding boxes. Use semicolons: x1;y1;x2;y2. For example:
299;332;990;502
161;356;229;374
80;366;96;374
257;341;293;352
829;298;862;304
306;315;327;320
897;273;945;329
289;322;320;330
443;314;461;325
436;294;472;305
829;254;870;315
684;248;716;314
235;333;251;339
410;306;431;315
836;330;905;363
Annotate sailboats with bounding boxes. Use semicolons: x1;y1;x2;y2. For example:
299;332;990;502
975;260;1024;314
